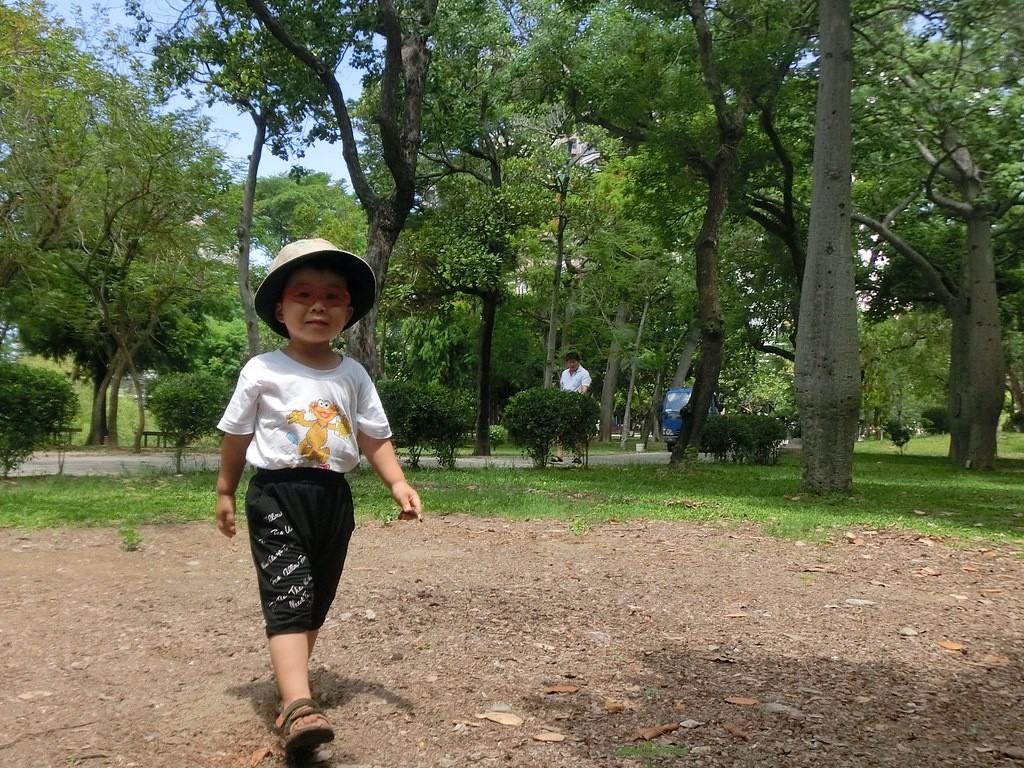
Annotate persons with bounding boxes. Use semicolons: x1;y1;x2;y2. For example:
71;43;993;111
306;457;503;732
213;238;423;761
547;350;593;465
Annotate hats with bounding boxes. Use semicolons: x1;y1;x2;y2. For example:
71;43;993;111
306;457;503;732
254;238;376;340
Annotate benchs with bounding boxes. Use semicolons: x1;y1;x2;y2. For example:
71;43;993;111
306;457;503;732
597;426;622;440
134;431;179;448
52;427;82;446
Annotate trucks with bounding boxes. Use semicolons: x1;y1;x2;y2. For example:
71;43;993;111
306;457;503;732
657;388;726;452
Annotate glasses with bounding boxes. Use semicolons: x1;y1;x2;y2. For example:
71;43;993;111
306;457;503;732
280;285;351;308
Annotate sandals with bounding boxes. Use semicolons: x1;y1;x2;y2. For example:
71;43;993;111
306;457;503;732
274;690;284;714
275;698;334;757
571;458;582;466
548;456;564;464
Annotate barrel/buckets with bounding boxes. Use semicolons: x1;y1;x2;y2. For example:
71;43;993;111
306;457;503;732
636;443;645;452
